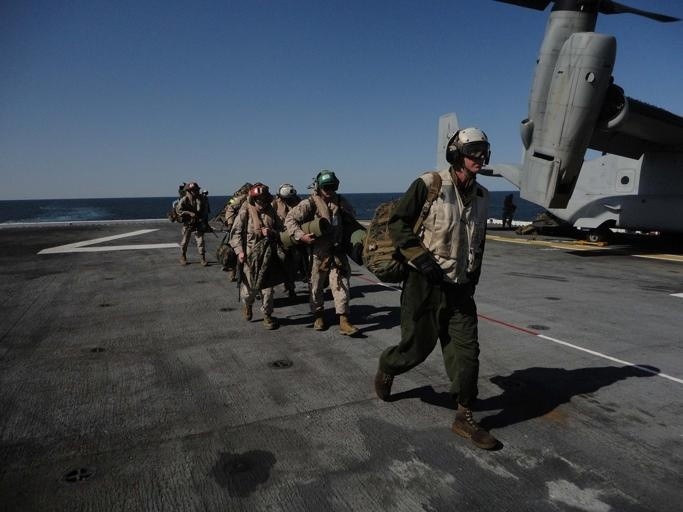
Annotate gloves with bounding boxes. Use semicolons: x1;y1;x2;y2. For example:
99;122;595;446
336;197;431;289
411;252;444;285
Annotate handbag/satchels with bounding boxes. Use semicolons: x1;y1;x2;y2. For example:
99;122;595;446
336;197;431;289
216;243;236;267
362;198;408;283
246;237;285;291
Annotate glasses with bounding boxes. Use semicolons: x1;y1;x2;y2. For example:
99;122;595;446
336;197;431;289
321;183;338;191
460;140;491;166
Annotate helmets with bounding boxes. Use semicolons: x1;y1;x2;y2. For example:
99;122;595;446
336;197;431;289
248;182;269;206
446;128;491;166
277;184;297;198
185;182;208;194
315;169;339;191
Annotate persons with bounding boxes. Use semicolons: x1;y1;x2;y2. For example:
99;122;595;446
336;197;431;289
503;193;517;229
166;169;368;337
374;127;502;452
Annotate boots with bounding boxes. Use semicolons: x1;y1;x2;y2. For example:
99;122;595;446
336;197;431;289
452;403;498;449
313;312;324;330
179;255;187;265
200;254;208;266
375;356;394;401
339;314;359;336
241;302;252;321
263;314;274;330
220;266;236;282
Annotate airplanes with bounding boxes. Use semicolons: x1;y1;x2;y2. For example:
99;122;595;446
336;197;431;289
431;1;682;244
